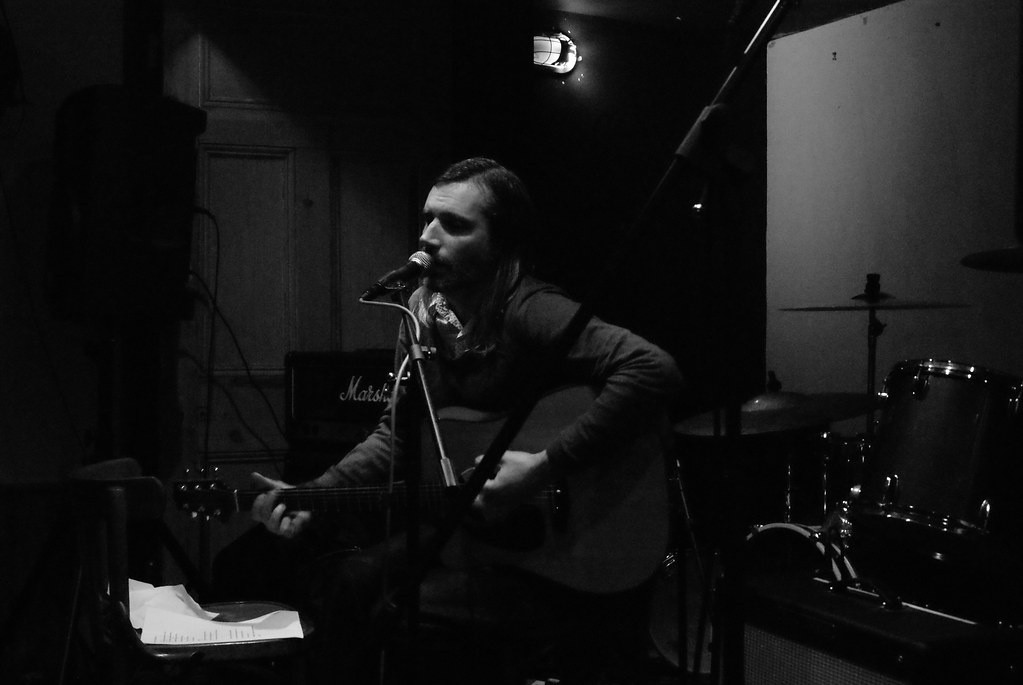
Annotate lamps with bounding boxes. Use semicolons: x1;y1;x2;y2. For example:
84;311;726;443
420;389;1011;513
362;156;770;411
534;33;577;73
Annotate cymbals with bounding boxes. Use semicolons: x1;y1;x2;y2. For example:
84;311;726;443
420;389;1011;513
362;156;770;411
671;390;874;438
778;291;973;312
960;244;1023;274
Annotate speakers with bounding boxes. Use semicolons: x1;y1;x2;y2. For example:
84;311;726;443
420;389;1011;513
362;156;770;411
281;349;410;494
46;82;206;321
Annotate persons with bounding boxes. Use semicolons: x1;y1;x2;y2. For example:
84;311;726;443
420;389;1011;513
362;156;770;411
249;156;679;685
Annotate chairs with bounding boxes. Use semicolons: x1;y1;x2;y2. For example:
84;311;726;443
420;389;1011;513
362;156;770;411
70;458;317;685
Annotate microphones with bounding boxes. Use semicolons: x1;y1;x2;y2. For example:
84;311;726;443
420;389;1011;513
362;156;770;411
360;252;434;303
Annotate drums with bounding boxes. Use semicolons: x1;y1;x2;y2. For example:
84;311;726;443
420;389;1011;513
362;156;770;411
854;358;1023;561
649;544;724;675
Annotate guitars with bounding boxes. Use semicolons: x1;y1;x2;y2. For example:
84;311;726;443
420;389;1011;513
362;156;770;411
170;381;675;598
